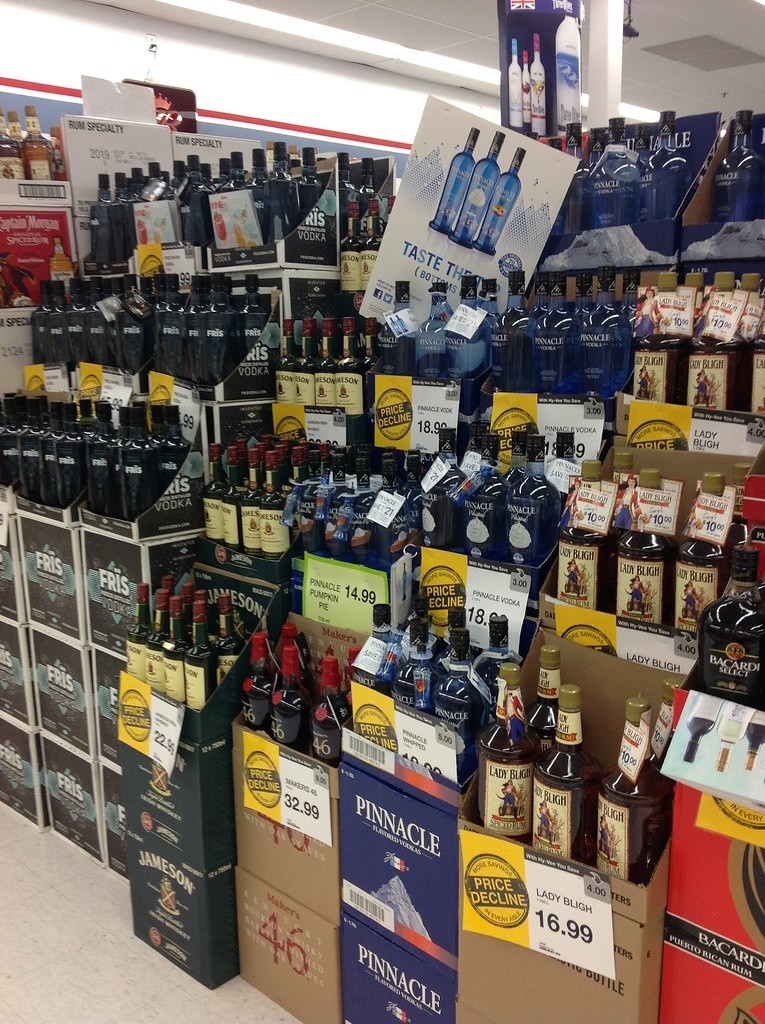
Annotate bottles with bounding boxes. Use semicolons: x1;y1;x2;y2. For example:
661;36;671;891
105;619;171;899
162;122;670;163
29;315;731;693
509;15;582;136
47;236;78;303
202;434;332;561
421;421;580;567
678;685;765;774
429;127;527;257
474;644;682;886
354;593;514;745
524;110;765;234
297;446;423;576
0;103;70;181
0;392;192;522
274;317;380;445
241;623;363;768
127;576;245;712
90;141;329;264
337;152;396;314
379;267;765;455
557;451;765;711
30;273;268;386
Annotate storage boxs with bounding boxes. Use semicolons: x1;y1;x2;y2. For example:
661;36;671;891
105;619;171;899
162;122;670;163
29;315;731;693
133;238;212;278
41;731;106;868
206;156;340;273
132;445;345;1024
0;618;40;731
224;271;342;357
95;650;125;773
80;531;205;663
159;291;283;403
0;515;28;626
78;403;211;543
83;251;135;278
205;398;277;456
0;716;49;828
99;763;130;885
16;515;88;645
29;626;98;760
12;487;88;527
345;105;765;1024
74;354;153;395
